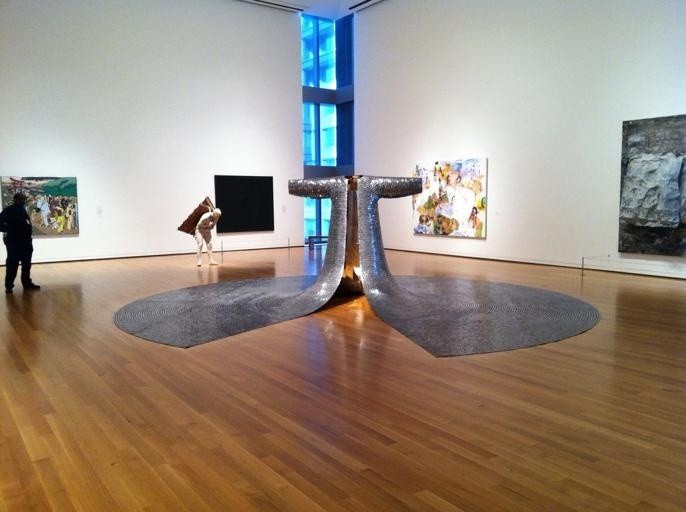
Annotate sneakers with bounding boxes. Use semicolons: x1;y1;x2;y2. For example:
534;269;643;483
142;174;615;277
23;283;41;288
6;287;12;292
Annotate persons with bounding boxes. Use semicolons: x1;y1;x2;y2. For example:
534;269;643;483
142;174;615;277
0;192;42;294
35;194;79;233
194;207;222;266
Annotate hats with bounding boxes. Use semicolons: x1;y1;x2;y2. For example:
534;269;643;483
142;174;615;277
13;193;29;199
